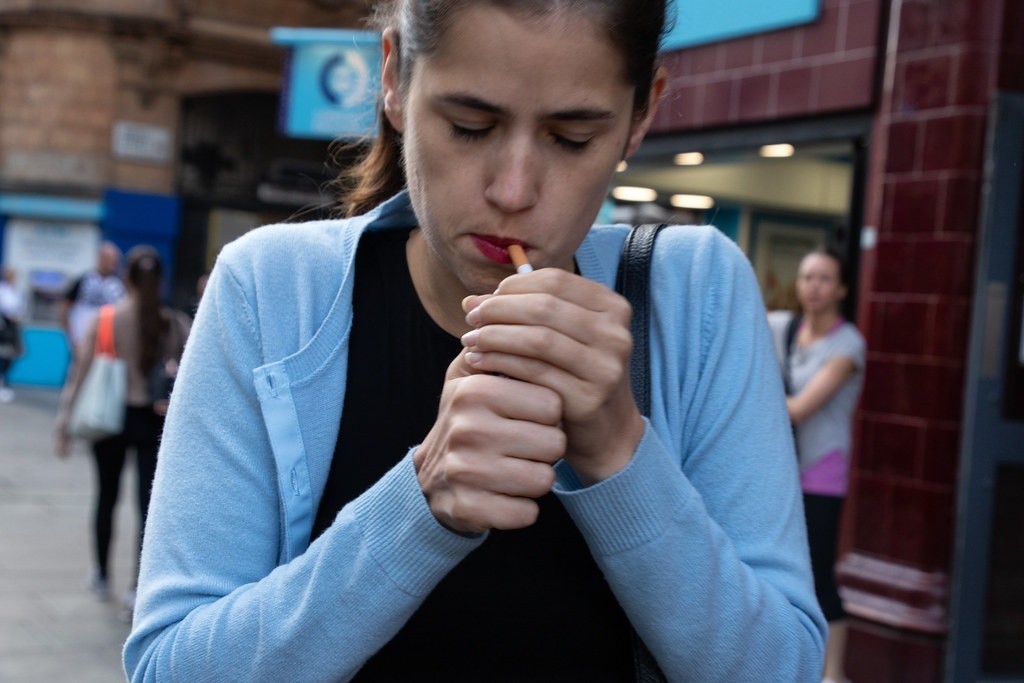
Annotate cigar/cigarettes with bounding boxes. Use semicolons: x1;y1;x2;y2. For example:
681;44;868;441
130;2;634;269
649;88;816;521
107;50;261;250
508;244;534;273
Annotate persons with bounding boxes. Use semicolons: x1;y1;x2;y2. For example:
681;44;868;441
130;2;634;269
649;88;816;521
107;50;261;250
767;243;867;683
54;239;194;624
0;264;29;404
121;0;829;683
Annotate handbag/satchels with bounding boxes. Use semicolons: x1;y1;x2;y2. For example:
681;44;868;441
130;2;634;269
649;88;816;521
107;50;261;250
65;303;126;440
145;317;184;415
